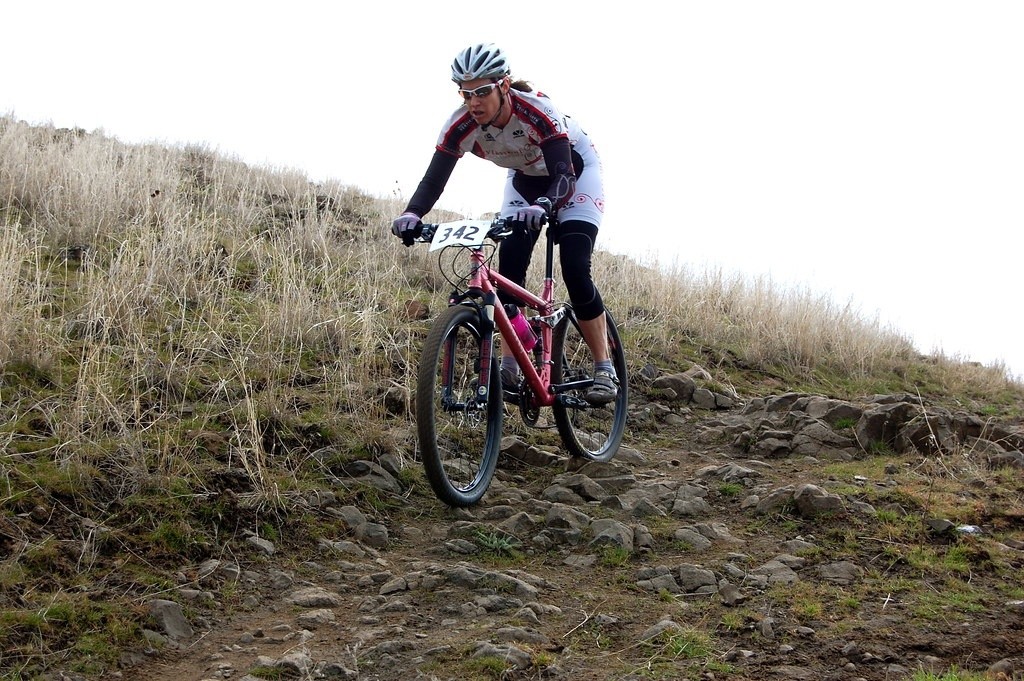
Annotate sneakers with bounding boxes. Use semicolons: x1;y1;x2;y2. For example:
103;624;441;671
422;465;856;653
470;367;520;392
585;367;619;404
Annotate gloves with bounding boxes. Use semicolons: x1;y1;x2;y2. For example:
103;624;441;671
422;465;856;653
391;212;424;247
511;204;549;234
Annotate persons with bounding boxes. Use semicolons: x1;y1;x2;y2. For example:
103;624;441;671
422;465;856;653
392;43;621;404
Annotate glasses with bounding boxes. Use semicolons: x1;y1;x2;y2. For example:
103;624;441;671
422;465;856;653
458;75;510;99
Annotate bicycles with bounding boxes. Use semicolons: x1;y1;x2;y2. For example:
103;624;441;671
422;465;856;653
390;211;632;510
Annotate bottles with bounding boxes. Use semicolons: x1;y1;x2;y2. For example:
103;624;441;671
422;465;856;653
504;304;539;351
531;325;542;354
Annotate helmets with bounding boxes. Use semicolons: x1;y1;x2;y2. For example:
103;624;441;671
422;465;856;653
452;43;509;84
